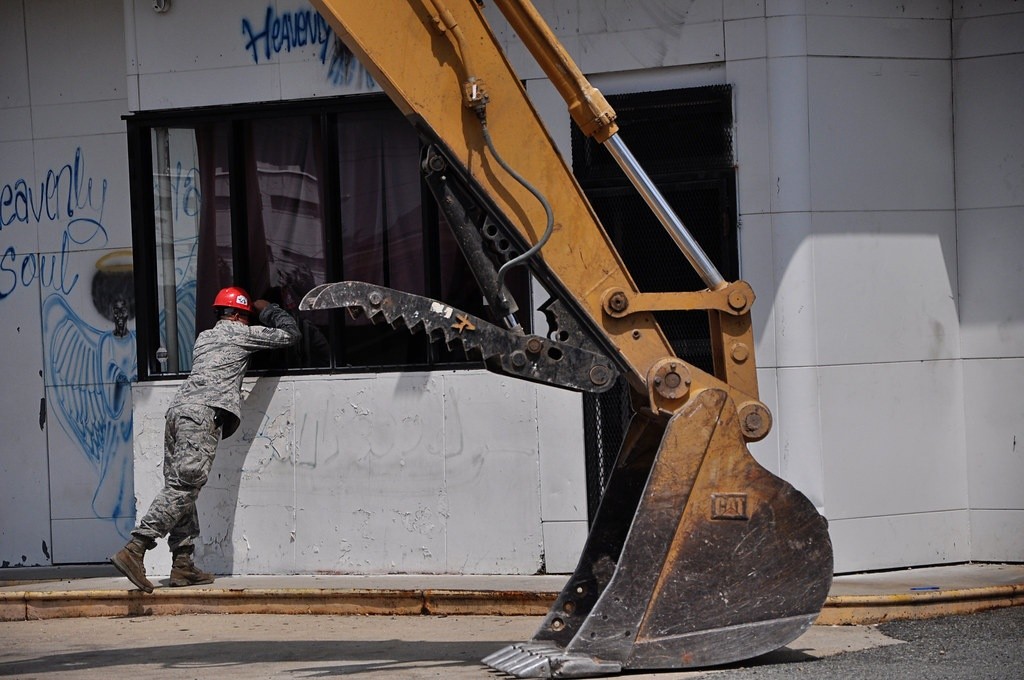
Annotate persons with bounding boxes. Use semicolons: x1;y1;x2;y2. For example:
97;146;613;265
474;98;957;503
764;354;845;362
110;285;304;594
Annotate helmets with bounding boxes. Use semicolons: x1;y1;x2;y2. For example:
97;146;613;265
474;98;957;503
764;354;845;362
213;287;253;311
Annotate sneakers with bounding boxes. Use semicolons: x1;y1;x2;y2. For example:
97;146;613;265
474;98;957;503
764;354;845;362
169;558;214;586
110;542;155;594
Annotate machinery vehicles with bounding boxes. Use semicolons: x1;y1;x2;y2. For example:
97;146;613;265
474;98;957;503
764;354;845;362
298;0;835;680
